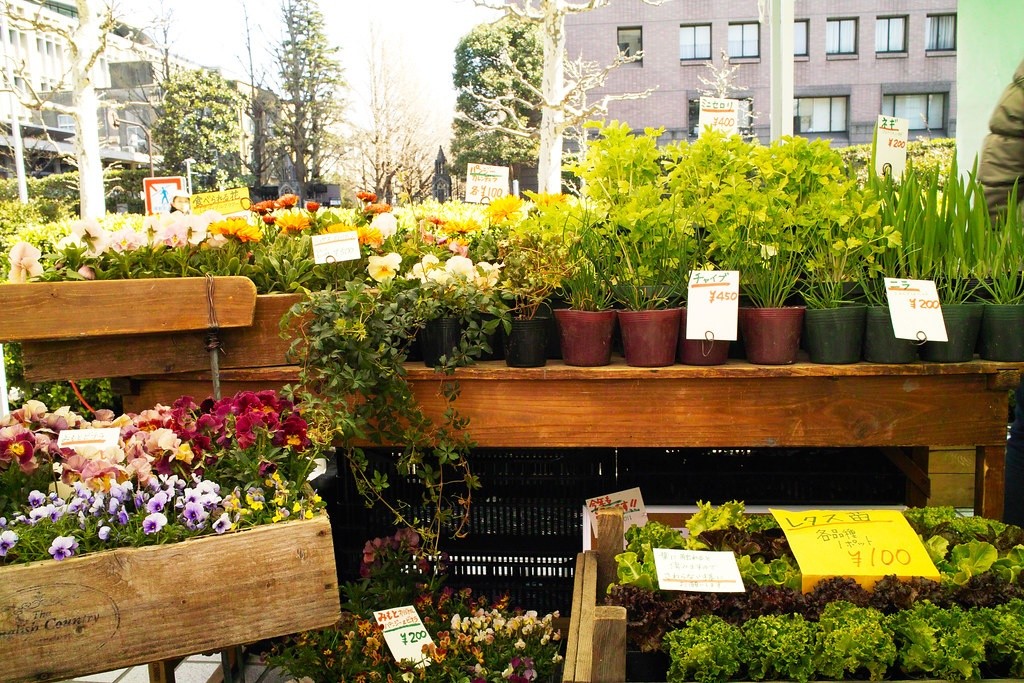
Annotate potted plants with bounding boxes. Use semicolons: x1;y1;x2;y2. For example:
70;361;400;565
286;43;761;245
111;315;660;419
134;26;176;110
276;119;1023;588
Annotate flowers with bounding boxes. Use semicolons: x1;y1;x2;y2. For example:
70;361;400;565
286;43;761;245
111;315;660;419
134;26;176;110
3;185;393;295
0;385;333;572
249;526;566;683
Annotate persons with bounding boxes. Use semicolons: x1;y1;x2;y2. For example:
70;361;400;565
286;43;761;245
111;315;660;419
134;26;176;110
168;189;191;216
975;54;1024;530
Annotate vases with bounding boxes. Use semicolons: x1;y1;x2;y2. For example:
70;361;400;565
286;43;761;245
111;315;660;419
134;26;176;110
0;506;342;683
